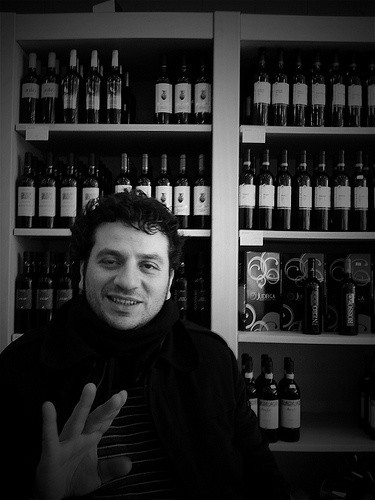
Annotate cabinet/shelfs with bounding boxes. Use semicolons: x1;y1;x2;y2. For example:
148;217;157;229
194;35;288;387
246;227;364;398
0;13;375;453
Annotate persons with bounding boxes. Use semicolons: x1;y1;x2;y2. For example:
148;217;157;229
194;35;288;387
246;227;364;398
0;189;302;500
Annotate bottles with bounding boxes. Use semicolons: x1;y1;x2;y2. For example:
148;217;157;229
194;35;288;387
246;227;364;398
155;54;212;124
21;47;132;124
14;151;211;229
240;147;375;232
301;257;322;335
13;251;210;332
247;47;374;128
337;256;359;336
241;352;300;443
359;354;375;439
